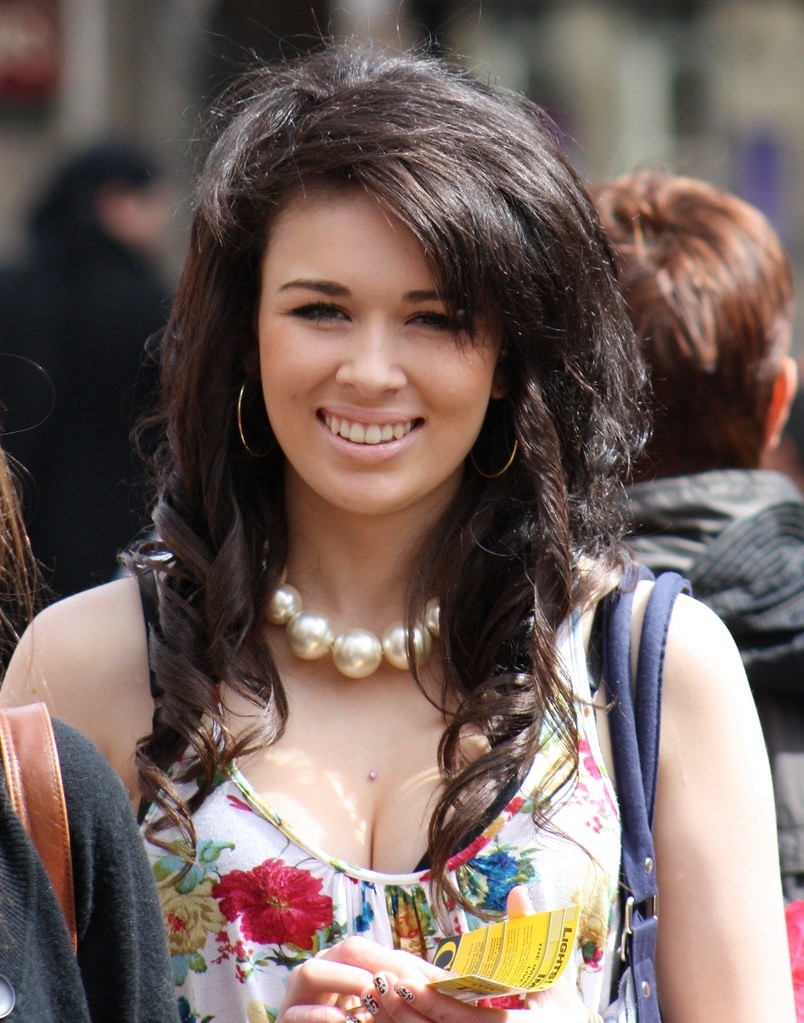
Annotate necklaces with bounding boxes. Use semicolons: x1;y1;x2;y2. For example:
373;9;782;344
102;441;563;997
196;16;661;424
243;530;470;678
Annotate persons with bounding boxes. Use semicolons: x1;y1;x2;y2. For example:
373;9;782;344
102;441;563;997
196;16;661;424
545;174;797;1006
0;701;176;1023
5;135;179;601
0;54;801;1023
